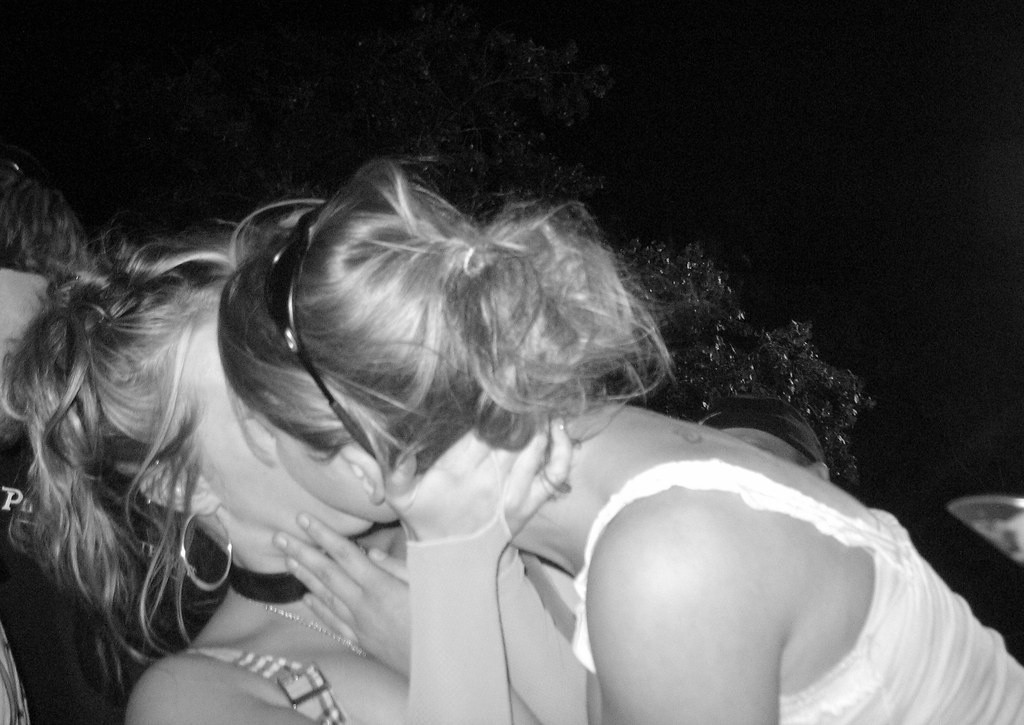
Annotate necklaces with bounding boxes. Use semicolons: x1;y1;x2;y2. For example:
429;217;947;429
227;561;309;604
238;594;368;657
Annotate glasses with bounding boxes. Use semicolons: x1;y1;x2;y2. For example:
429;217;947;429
262;201;381;462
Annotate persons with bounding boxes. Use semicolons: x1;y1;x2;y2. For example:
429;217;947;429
0;156;1024;724
